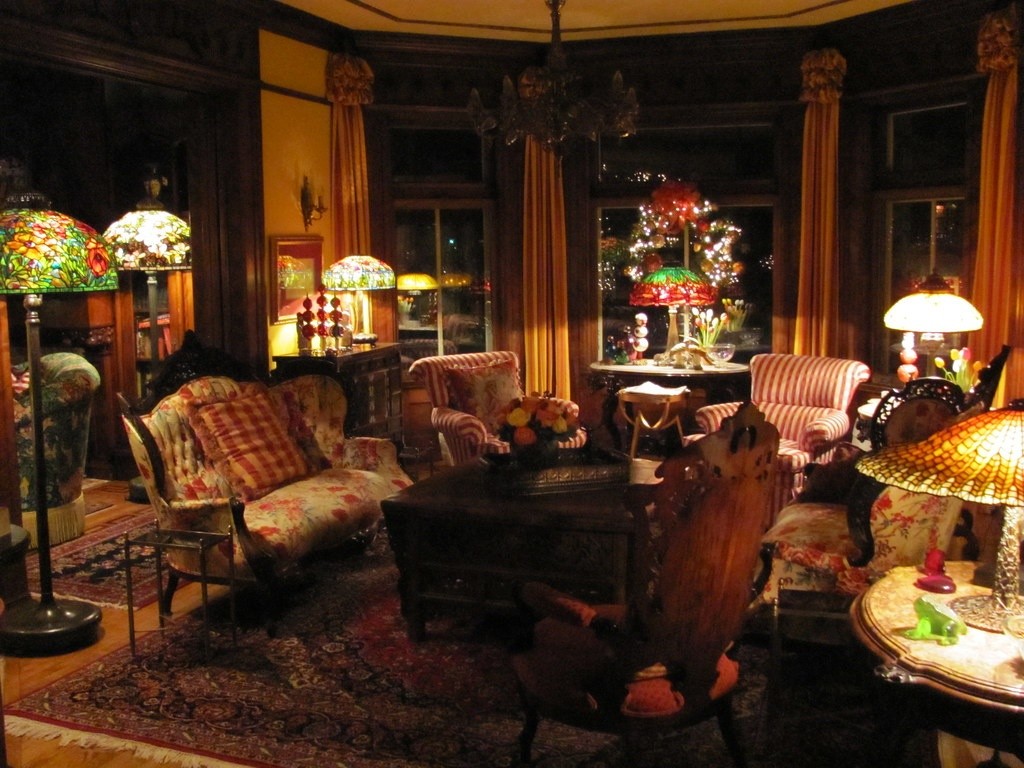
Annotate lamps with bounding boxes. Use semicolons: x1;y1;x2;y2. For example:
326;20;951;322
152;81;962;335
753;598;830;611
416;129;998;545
396;272;437;296
0;190;121;654
855;396;1024;636
320;255;395;348
466;0;642;154
884;268;984;377
300;174;328;231
629;261;720;369
101;165;193;505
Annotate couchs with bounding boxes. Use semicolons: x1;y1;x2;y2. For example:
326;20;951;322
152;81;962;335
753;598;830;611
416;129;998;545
408;351;592;467
0;353;99;550
683;353;870;506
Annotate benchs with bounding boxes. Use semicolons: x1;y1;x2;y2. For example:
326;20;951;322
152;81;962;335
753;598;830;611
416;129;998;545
746;400;985;696
117;328;418;637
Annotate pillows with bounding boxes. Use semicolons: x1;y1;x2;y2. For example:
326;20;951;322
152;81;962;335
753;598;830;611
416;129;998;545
187;382;329;502
442;359;526;435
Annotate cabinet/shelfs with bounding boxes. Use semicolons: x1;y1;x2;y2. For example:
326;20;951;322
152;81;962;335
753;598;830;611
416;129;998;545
270;344;408;456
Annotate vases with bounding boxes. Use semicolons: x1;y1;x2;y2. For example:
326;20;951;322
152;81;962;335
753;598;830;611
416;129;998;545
515;444;556;467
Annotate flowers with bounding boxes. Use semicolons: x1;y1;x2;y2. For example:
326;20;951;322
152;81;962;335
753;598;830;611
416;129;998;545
494;391;584;448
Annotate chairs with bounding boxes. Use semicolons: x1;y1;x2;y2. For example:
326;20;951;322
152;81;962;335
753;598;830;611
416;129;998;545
869;344;1013;453
509;398;794;768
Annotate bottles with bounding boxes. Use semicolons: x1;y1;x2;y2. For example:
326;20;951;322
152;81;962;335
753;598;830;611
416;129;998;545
605;326;637;363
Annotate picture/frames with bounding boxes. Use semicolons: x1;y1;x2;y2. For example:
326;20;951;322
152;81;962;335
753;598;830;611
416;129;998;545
269;234;324;326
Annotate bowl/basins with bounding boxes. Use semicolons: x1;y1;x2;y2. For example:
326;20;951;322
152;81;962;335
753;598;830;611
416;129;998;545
709;342;736;362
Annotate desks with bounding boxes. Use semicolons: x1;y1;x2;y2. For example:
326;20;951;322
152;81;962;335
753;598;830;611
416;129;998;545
587;360;751;457
124;518;237;667
377;450;652;642
848;560;1024;768
